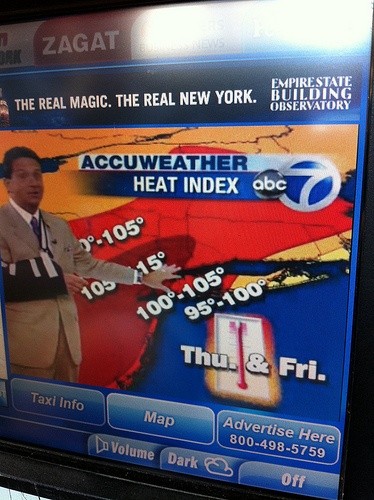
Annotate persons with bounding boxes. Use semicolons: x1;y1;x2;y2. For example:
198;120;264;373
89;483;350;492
2;145;182;381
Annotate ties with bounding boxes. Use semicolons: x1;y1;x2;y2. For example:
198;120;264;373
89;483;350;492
31;216;41;239
